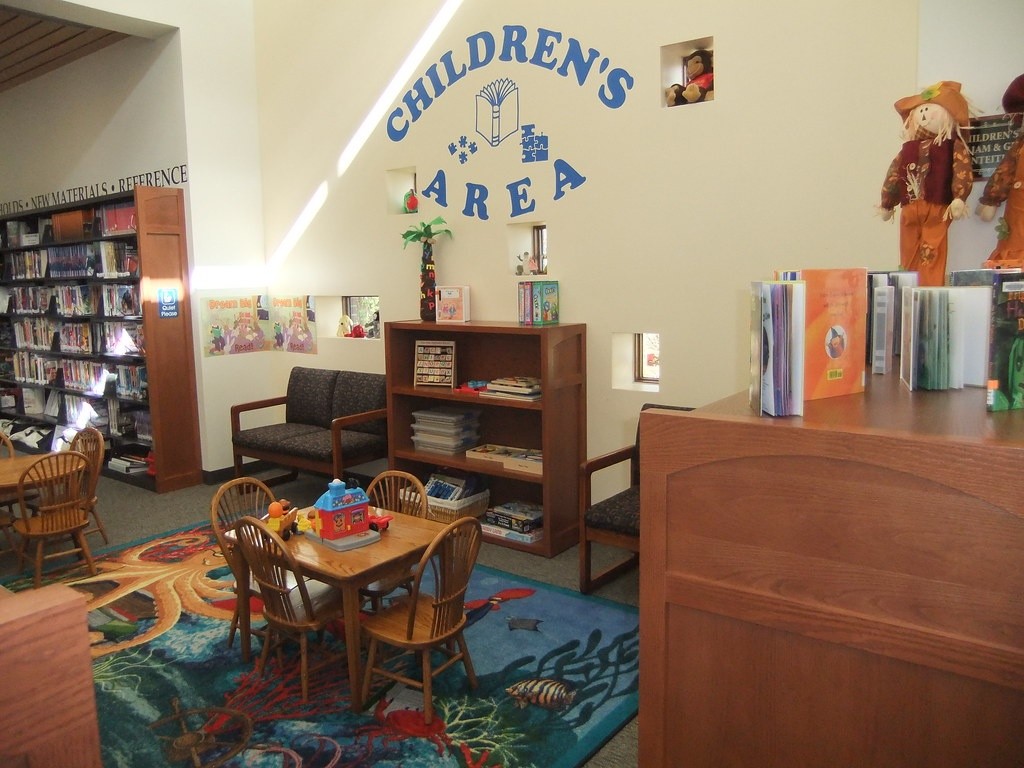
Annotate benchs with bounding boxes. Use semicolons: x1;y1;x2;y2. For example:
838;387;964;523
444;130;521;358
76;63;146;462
228;365;387;496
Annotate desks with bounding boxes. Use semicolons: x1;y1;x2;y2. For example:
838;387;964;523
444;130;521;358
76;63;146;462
215;504;456;701
0;452;87;554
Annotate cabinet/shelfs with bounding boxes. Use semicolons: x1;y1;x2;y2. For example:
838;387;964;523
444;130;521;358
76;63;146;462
0;184;202;497
634;366;1024;768
365;317;594;561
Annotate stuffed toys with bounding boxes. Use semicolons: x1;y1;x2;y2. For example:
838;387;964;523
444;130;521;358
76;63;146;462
337;310;379;338
665;49;714;107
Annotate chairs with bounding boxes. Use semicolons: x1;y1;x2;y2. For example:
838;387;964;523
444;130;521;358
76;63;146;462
0;428;37;548
233;513;367;707
24;424;110;556
356;513;485;730
208;476;311;668
349;468;434;614
0;504;16;563
16;448;97;591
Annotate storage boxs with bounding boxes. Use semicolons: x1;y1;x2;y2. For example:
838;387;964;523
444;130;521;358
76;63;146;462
101;198;135;235
52;206;89;239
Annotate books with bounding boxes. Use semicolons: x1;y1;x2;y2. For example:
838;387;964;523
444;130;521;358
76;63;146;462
0;201;153;475
422;473;465;501
748;268;1024;417
479;376;540;402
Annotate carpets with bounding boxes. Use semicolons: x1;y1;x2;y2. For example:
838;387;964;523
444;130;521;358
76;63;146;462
0;500;640;768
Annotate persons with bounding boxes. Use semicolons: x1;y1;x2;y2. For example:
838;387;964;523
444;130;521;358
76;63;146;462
879;81;973;286
975;73;1024;271
269;499;304;540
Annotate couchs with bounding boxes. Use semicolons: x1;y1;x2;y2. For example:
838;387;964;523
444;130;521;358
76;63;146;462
575;403;696;600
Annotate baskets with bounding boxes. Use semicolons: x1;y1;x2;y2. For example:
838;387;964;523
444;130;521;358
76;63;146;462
399;486;490;523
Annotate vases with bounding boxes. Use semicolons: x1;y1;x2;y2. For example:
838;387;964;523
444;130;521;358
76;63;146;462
418;237;436;320
